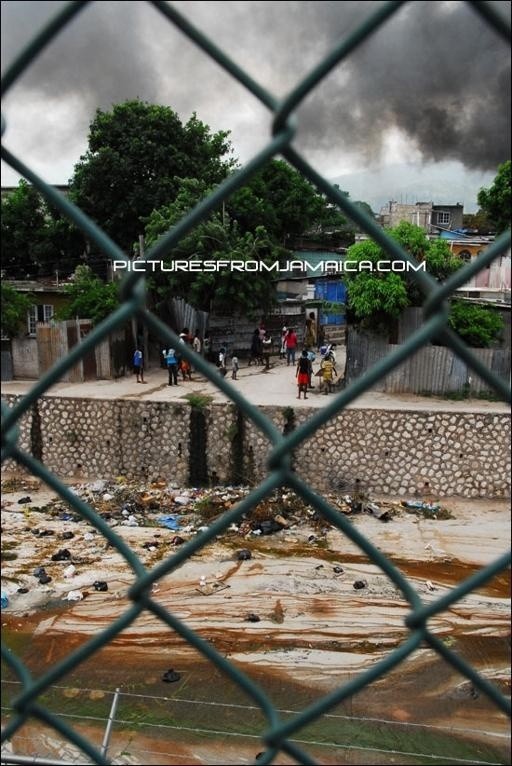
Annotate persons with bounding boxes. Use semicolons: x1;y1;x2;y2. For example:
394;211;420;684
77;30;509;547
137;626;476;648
250;319;339;399
161;326;239;386
131;344;149;384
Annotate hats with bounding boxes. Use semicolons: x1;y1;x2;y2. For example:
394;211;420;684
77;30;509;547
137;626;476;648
179;332;187;337
